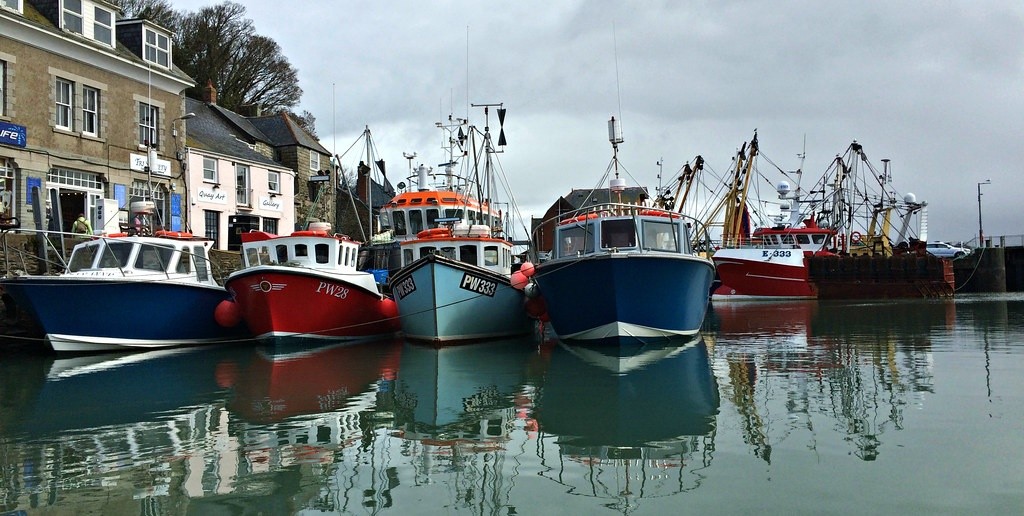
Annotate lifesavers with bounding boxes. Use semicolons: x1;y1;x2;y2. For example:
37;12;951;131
641;210;679;218
850;232;861;242
417;228;449;239
109;233;127;237
561;214;598;224
291;231;327;236
155;231;192;237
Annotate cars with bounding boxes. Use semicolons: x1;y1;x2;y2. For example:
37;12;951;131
924;241;971;258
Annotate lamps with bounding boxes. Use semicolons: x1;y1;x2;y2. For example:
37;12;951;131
213;185;219;189
270;195;276;197
173;112;195;137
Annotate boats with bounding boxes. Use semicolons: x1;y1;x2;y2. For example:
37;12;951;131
384;219;536;346
530;115;717;345
712;207;846;302
226;226;397;346
0;131;238;353
364;85;504;292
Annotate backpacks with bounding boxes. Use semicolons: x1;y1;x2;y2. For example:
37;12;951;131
77;219;87;233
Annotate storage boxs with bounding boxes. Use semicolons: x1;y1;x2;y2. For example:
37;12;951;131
365;269;389;284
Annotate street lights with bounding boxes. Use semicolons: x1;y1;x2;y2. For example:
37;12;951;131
977;182;991;248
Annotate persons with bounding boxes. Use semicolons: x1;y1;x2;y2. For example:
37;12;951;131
133;215;140;234
71;214;94;239
